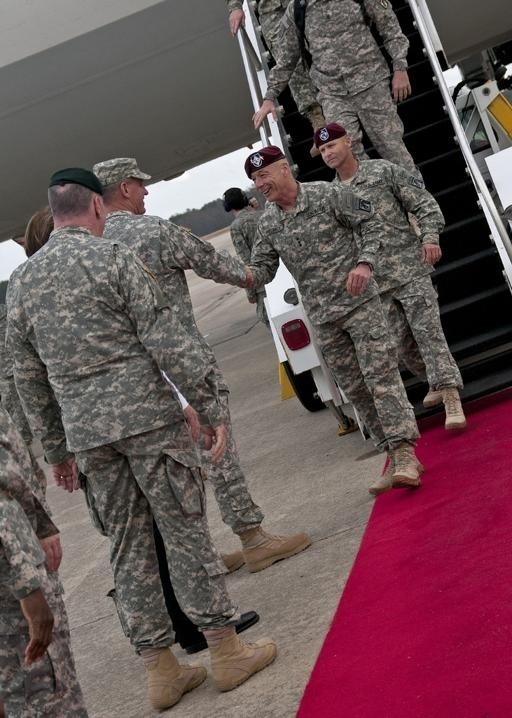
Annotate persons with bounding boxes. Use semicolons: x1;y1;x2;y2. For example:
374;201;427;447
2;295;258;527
6;169;279;710
0;304;88;718
312;124;466;431
225;0;327;158
87;157;311;574
222;187;267;327
251;0;425;236
245;146;425;496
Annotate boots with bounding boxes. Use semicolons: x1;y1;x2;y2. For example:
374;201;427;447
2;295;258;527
308;107;326;159
367;449;396;495
442;386;467;430
138;644;206;710
393;445;424;488
219;550;248;575
203;625;277;694
239;525;313;574
423;390;443;408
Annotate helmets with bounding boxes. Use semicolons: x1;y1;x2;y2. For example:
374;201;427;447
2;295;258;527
222;188;248;212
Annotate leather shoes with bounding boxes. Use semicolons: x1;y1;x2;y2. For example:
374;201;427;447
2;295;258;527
184;610;259;654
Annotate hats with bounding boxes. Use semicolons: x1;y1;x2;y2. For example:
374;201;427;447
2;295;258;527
48;167;104;195
93;157;152;186
244;146;285;178
314;123;347;149
